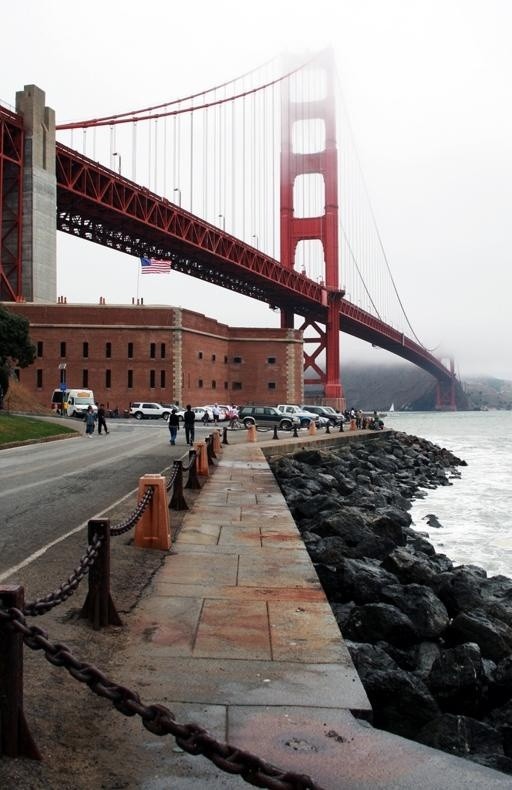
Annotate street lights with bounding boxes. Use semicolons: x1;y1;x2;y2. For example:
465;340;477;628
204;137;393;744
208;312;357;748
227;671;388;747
317;274;394;328
57;362;66;415
252;234;258;250
173;188;181;207
111;151;122;175
299;264;306;272
217;214;225;231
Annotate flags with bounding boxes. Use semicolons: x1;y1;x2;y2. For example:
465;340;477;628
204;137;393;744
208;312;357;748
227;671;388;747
141;258;172;274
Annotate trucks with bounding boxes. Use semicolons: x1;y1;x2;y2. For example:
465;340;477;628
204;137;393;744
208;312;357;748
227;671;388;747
49;387;99;418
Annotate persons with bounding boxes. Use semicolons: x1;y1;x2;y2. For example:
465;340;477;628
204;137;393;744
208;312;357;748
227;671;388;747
344;407;384;430
168;403;238;445
64;400;109;439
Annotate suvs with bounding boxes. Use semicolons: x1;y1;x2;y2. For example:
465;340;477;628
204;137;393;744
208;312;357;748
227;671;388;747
127;401;172;421
238;403;346;431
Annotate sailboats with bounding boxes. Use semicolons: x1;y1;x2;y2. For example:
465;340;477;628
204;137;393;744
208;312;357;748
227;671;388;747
389;402;395;412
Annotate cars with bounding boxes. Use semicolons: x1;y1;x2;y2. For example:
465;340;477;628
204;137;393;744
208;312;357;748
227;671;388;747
160;403;239;423
